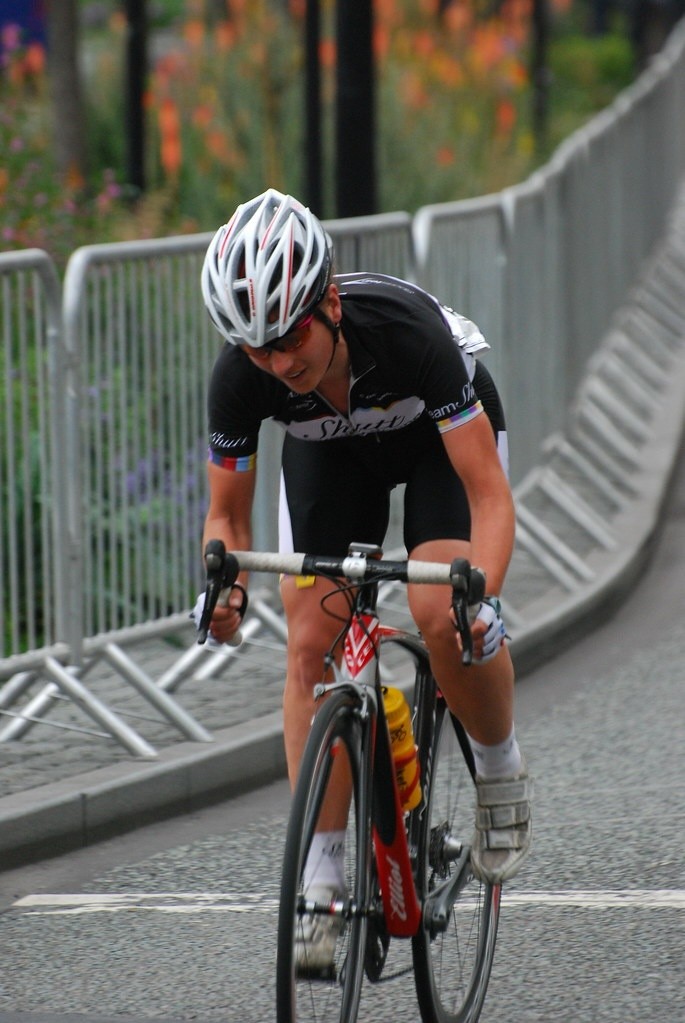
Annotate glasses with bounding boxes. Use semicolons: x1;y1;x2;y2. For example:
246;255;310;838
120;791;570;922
240;297;324;359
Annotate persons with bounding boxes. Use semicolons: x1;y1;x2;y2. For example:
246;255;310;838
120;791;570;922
191;188;535;983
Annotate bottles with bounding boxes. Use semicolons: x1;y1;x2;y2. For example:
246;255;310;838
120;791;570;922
381;686;421;813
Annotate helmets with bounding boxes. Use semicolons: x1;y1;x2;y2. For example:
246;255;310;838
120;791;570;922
199;187;333;349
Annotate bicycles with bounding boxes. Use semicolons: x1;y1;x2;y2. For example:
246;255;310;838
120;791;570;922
192;538;507;1022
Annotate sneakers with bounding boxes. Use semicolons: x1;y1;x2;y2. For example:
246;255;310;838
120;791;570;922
294;885;349;978
471;749;532;883
